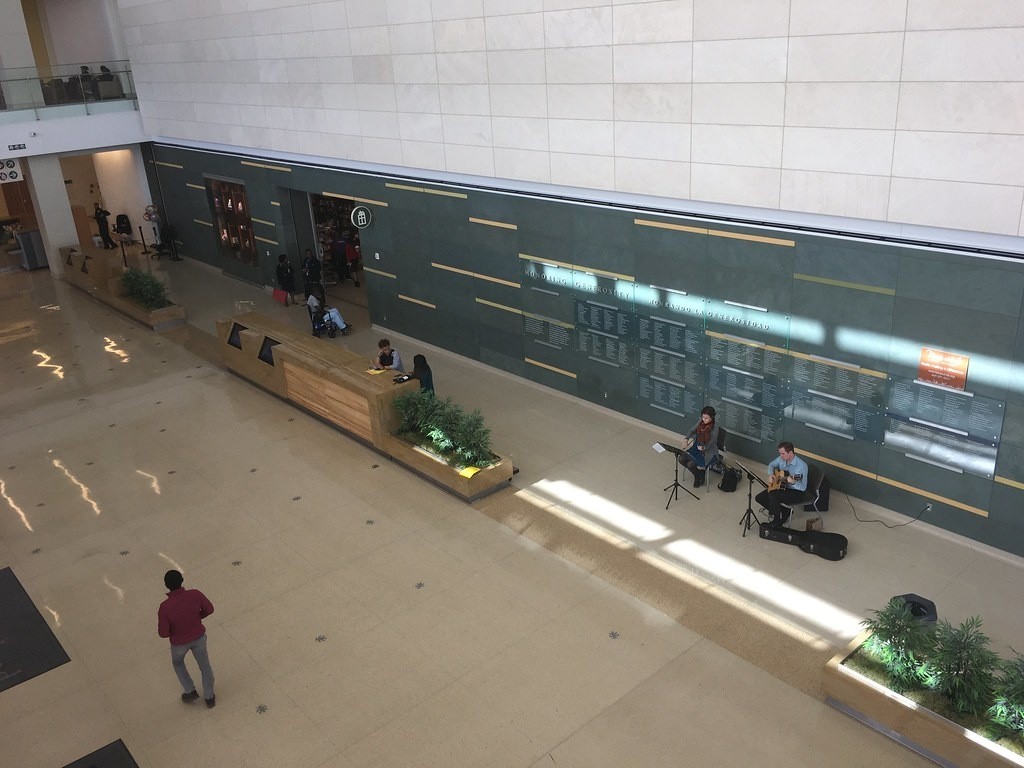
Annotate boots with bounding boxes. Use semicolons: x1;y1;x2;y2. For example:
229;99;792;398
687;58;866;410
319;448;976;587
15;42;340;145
354;280;360;287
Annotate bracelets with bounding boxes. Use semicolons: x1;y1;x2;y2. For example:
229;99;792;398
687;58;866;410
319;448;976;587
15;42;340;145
383;365;385;370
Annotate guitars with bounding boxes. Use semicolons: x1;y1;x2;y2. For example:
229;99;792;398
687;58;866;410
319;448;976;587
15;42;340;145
768;468;803;492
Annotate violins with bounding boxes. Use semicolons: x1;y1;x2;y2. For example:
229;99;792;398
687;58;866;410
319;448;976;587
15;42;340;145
697;422;714;457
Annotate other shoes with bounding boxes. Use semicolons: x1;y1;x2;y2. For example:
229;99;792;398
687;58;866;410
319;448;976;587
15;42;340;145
205;694;216;709
110;244;118;249
341;328;350;332
700;470;705;486
694;474;700;488
346;324;353;327
182;690;199;701
768;520;783;529
292;302;299;305
285;302;289;307
104;245;109;249
781;508;793;524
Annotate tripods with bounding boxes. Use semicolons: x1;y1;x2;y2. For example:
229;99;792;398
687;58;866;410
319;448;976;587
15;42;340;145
735;461;770;537
657;442;700;509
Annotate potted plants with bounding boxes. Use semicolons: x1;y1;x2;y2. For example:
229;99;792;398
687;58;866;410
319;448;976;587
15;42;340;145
823;595;1024;768
118;264;186;330
390;385;513;504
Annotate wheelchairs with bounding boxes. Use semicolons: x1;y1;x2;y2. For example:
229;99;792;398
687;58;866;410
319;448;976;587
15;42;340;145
300;297;352;339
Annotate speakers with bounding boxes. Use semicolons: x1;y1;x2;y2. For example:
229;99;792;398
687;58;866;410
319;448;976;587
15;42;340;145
803;477;830;512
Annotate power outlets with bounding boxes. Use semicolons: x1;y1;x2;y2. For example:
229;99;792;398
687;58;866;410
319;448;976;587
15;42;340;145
926;503;933;512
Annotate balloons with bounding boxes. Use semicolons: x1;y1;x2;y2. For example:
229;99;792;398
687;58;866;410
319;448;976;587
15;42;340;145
143;206;161;223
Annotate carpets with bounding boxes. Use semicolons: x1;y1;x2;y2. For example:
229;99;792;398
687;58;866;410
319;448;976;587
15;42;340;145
62;737;140;768
0;566;71;694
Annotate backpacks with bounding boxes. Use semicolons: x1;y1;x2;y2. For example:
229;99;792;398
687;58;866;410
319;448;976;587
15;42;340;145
718;468;737;492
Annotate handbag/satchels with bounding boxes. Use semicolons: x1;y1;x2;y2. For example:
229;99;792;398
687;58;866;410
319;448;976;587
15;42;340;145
272;288;288;303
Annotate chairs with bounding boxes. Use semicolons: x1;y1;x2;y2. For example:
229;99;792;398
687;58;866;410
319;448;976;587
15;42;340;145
683;426;728;492
780;462;825;530
39;74;123;104
149;227;173;260
113;215;135;246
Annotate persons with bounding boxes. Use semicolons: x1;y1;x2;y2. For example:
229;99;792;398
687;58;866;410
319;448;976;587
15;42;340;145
90;202;118;250
300;248;322;306
276;254;299;306
755;441;808;530
678;406;719;488
346;230;361;287
157;570;216;709
80;66;92;81
408;353;435;398
95;66;113;82
375;338;406;374
307;286;352;332
